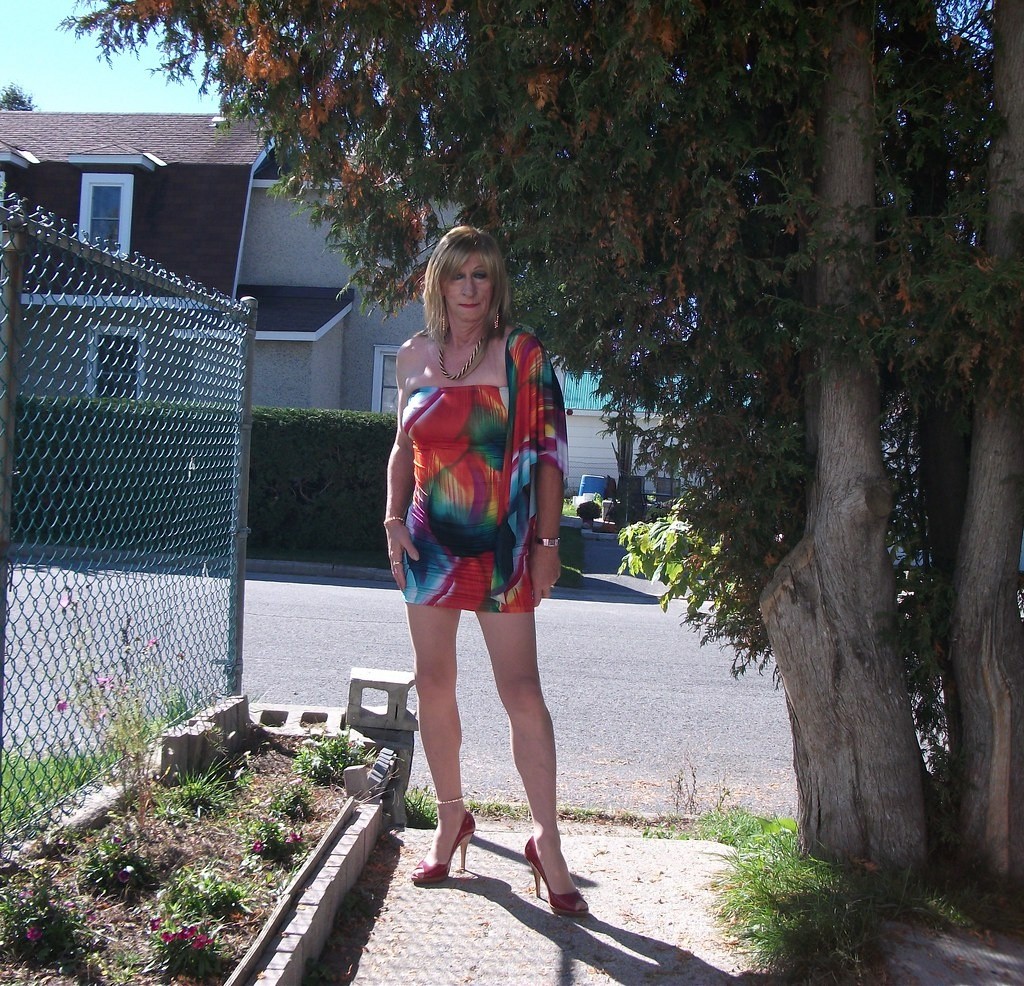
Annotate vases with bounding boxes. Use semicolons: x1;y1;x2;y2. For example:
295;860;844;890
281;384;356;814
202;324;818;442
582;519;594;529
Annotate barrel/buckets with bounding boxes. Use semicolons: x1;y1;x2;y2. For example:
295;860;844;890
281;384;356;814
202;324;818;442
578;475;606;500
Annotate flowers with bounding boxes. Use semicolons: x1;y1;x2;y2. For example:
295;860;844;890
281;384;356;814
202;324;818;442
576;500;602;519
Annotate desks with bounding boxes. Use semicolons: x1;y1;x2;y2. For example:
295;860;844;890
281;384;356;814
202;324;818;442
642;493;671;500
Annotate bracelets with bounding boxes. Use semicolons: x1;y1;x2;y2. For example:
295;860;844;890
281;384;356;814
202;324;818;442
383;516;406;527
535;533;561;548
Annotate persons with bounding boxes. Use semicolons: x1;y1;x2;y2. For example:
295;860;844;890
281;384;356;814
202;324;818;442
382;225;592;921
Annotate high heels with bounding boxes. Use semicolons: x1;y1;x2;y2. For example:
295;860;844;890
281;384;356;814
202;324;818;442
412;809;476;883
524;835;589;915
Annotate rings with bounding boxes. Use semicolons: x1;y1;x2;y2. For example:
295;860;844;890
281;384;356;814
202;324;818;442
550;585;554;588
393;561;402;567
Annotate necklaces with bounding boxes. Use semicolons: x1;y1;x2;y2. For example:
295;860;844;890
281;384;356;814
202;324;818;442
438;326;490;380
